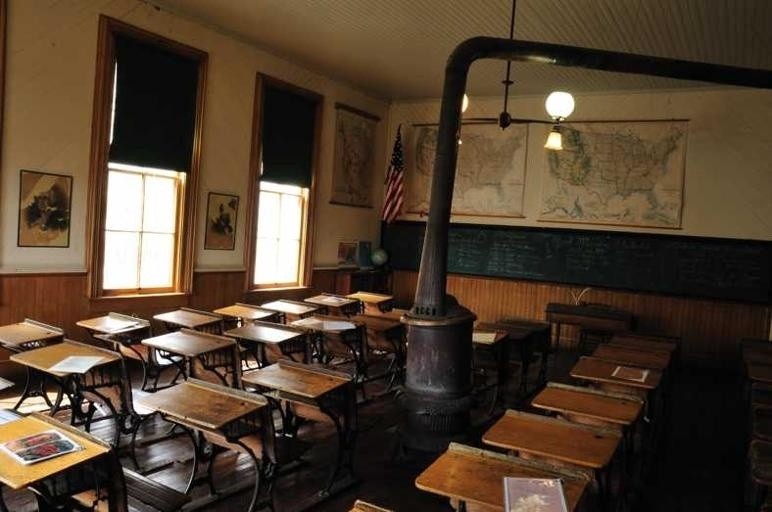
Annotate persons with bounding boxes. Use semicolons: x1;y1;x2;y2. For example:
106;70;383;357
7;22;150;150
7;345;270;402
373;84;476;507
28;184;67;231
212;204;233;247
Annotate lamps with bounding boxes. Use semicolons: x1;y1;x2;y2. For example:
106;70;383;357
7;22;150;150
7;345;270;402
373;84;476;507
543;91;576;153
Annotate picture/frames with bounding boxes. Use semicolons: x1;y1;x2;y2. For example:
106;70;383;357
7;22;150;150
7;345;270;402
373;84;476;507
204;190;240;251
18;169;74;249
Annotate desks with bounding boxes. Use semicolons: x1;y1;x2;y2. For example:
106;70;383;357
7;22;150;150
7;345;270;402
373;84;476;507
469;313;553;414
333;268;387;295
738;336;771;510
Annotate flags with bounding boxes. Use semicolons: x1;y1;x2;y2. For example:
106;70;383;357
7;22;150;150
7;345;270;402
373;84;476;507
380;133;405;224
